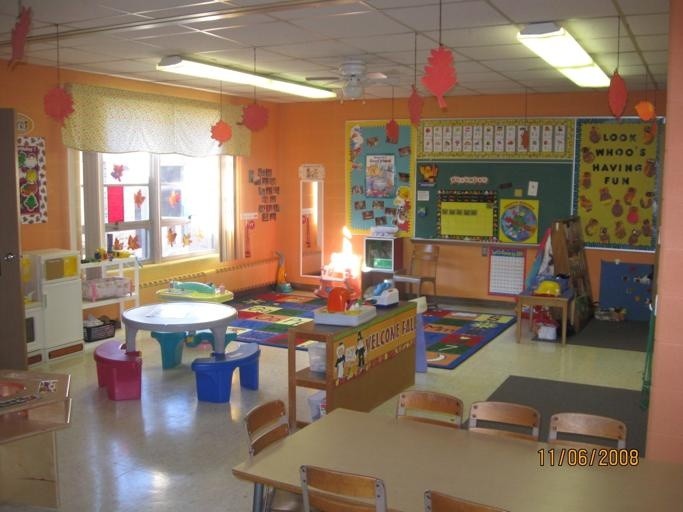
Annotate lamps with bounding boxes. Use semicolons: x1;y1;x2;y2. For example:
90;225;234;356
154;55;337;99
516;19;612;88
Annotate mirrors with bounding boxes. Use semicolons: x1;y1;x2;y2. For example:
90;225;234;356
300;178;324;278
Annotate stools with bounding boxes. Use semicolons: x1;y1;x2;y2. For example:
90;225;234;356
517;287;578;347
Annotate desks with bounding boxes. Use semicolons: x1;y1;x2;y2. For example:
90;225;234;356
156;279;234;303
121;302;239;354
232;407;683;511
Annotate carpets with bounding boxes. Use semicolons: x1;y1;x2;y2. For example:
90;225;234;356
457;375;648;459
530;315;652;352
174;280;517;370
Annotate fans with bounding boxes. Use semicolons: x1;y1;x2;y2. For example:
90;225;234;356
305;62;407;101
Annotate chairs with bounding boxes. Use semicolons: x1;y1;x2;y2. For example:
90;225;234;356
468;402;543;442
548;412;627;455
300;464;388;512
395;390;463;430
423;489;511;512
244;399;319;512
390;244;439;308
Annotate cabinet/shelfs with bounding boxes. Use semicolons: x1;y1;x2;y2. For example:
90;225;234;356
82;256;140;349
24;248;84;361
0;369;72;511
24;302;44;365
523;215;595;334
361;236;403;300
287;301;416;431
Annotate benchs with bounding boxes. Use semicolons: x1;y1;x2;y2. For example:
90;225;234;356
151;328;237;371
190;343;261;403
93;340;143;401
